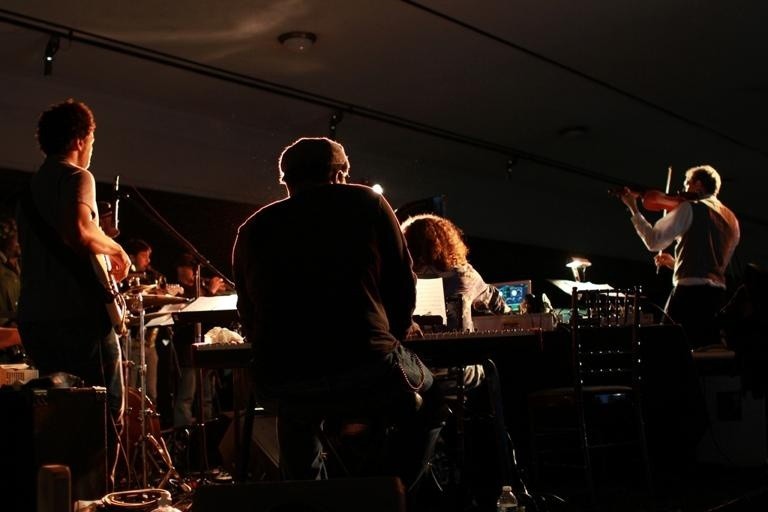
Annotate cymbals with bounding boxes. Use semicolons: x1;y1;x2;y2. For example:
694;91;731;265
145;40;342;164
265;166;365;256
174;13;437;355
119;295;189;305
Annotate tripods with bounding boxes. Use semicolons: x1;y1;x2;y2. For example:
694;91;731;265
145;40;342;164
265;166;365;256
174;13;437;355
125;309;173;489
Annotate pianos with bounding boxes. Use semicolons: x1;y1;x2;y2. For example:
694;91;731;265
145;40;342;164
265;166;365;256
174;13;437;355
192;314;554;367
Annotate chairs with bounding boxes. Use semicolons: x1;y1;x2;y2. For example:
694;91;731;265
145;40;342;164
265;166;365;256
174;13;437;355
527;285;654;506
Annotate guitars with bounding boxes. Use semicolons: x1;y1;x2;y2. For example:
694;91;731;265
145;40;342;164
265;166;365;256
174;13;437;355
92;250;130;336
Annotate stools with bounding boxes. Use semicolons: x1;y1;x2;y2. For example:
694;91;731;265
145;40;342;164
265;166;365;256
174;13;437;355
431;394;468;475
285;401;368;480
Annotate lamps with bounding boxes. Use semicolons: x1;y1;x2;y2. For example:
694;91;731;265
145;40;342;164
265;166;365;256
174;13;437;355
278;32;316;53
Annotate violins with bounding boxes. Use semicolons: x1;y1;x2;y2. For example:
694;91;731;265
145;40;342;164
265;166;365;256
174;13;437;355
608;188;685;212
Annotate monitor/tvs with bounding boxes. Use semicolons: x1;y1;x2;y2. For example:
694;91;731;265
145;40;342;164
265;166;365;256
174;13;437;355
492;280;532;314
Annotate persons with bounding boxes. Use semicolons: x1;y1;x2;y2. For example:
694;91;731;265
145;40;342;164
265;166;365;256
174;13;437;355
16;99;129;496
618;164;741;331
405;202;527;479
233;138;457;512
0;217;27;327
120;228;235;488
